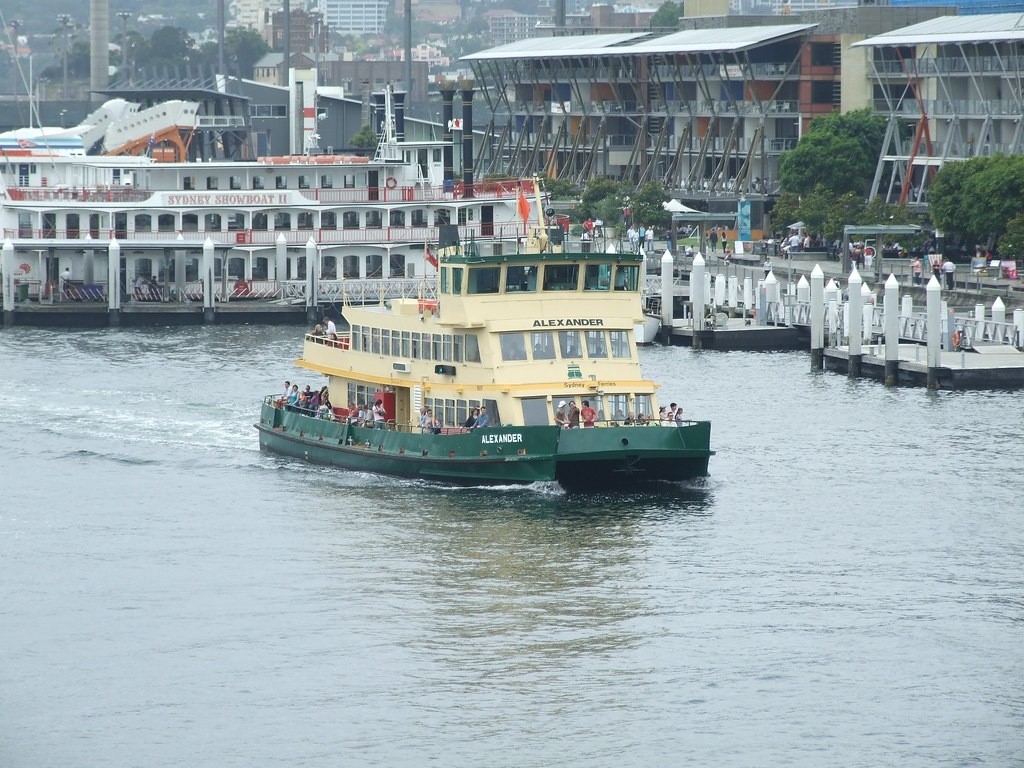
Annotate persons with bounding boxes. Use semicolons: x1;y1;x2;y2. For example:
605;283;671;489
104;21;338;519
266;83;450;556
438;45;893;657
720;232;727;253
912;257;921;286
60;267;71;292
753;176;766;189
852;244;873;270
976;248;992;258
553;400;604;428
583;217;603;239
615;403;684;427
666;229;672;250
416;406;434;433
508;342;554;360
568;344;577;359
279;381;327;417
614;266;628;290
595;347;602;358
347;399;386;429
942;258;956;290
780;232;811;252
710;229;718;252
932;261;939;277
459;407;489;430
686;245;693;257
627;225;653;254
135;274;157;294
313;316;338;347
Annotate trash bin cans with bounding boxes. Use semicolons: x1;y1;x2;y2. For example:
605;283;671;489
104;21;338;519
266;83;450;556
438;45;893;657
16;283;29;300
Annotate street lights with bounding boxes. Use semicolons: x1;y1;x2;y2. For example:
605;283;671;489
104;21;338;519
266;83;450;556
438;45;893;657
55;12;75;100
10;18;24;95
115;8;134;82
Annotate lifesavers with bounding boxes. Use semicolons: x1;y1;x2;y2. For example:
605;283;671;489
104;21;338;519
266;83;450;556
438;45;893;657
952;332;959;346
754;308;757;319
386;177;397;189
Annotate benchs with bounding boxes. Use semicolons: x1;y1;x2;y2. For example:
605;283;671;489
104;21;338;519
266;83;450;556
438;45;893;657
440;427;467;434
333;407;349;424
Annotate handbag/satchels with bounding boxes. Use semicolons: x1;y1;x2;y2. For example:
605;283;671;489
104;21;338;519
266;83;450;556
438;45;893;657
379;412;385;415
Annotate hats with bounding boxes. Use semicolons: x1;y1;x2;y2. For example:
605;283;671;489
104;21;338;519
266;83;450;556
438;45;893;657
658;406;667;413
558;401;566;407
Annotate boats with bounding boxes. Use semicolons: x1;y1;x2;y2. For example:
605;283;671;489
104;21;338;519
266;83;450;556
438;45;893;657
252;170;719;489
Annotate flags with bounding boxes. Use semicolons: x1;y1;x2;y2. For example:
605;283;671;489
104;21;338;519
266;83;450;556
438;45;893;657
426;249;438;267
518;194;530;222
